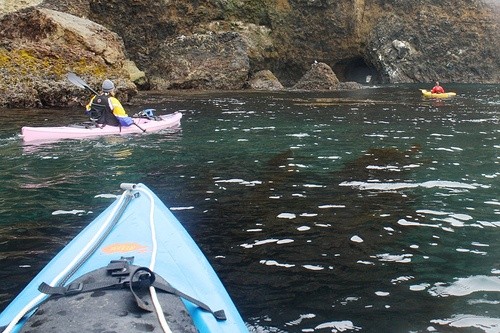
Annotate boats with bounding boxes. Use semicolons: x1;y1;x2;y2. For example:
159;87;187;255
21;110;184;143
0;179;250;330
422;89;456;98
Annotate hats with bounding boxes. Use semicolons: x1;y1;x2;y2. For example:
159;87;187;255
102;80;114;92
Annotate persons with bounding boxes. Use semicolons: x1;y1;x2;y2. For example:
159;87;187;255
86;79;133;127
431;81;444;93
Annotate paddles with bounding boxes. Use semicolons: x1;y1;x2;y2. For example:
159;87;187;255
418;89;422;91
65;72;147;133
